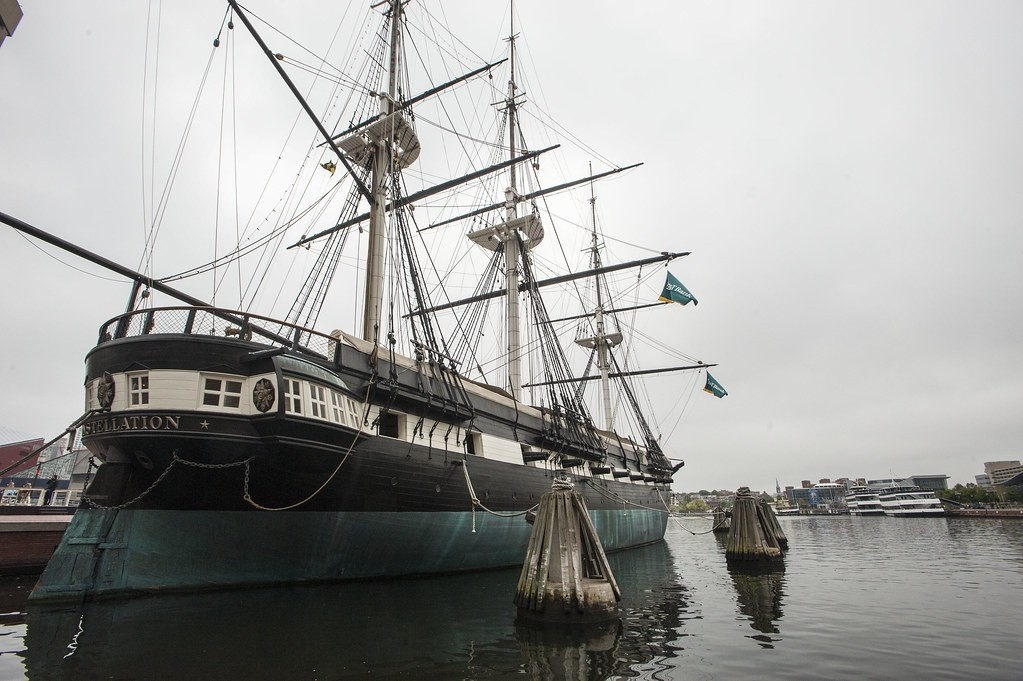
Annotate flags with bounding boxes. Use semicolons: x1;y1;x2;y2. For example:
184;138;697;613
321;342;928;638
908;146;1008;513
702;370;728;398
658;271;698;306
320;161;336;176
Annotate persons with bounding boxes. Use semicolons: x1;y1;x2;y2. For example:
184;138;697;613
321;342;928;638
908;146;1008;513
43;475;57;505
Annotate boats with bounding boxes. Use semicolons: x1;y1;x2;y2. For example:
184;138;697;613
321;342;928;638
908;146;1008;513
878;468;945;518
775;505;800;516
844;480;885;516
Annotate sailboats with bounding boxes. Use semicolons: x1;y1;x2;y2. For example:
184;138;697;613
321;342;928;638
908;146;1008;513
0;0;730;614
